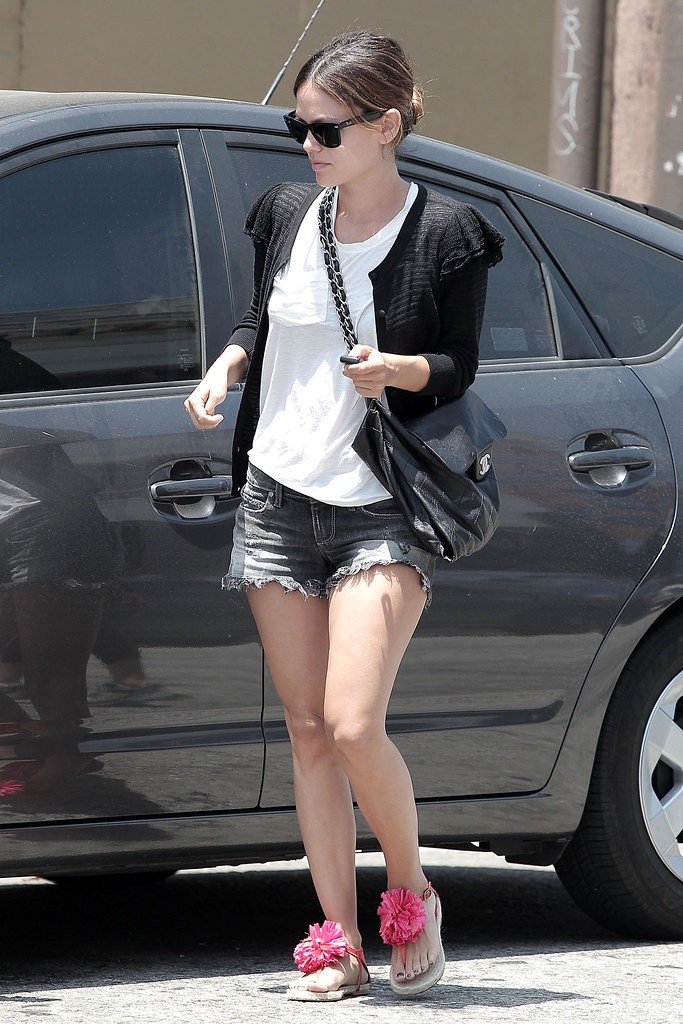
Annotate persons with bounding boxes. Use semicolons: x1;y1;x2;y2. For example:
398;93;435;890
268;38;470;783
186;35;507;1000
1;346;159;800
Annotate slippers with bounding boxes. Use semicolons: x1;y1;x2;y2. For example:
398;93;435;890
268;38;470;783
85;680;162;705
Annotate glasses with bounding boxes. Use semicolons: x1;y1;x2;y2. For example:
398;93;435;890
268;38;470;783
283;107;383;149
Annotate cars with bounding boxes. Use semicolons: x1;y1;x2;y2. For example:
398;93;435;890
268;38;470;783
0;90;683;942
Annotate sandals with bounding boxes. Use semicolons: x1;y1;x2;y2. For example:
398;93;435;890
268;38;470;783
1;756;76;802
287;922;372;1002
379;882;445;995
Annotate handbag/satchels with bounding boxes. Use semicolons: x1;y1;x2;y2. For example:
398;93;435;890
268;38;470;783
350;391;507;559
